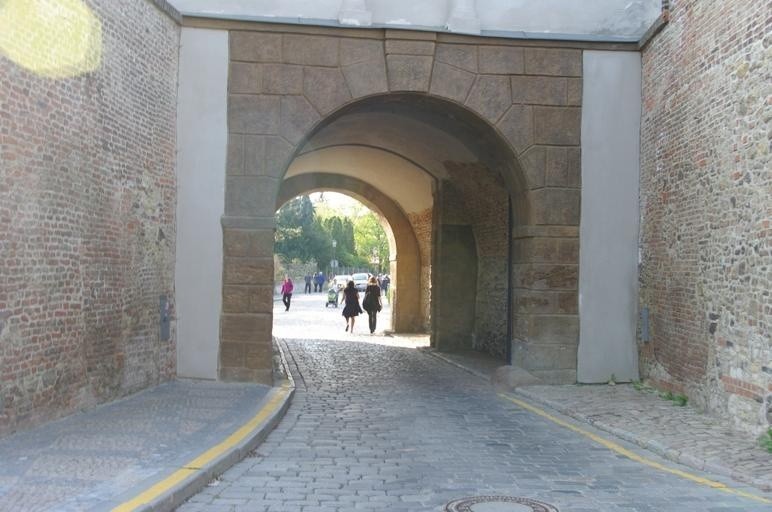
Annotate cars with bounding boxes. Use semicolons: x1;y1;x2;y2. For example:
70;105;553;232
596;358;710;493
351;272;372;290
328;276;352;289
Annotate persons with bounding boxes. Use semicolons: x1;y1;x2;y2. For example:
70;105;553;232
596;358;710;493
376;275;390;296
318;272;324;292
281;275;293;311
331;278;339;308
341;281;360;332
312;271;318;292
304;271;312;294
363;277;382;333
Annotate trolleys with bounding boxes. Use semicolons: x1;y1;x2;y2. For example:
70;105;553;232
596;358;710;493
326;288;339;308
